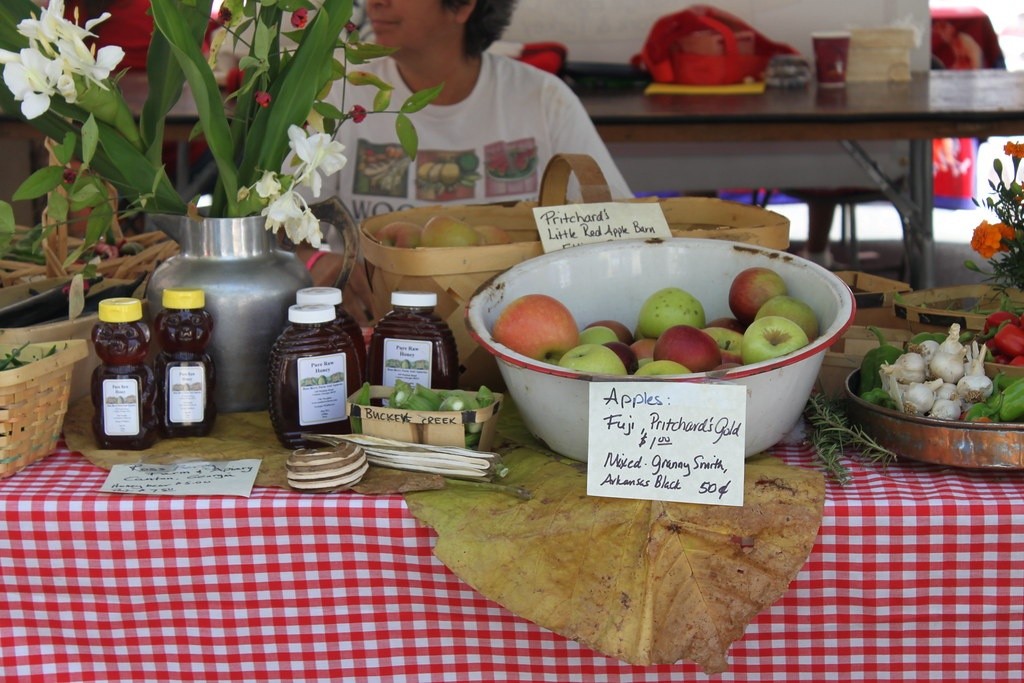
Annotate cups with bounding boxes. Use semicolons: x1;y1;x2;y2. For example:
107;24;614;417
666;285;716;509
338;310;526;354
812;32;850;89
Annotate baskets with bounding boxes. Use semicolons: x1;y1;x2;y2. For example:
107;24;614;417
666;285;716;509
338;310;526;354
0;337;88;478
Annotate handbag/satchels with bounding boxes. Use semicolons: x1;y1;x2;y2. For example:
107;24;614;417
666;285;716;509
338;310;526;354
630;4;801;84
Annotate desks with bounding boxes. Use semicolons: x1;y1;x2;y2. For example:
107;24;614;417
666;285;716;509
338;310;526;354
0;71;1023;289
632;5;1007;211
0;384;1024;683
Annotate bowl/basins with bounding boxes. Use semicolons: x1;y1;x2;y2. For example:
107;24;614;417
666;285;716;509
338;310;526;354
465;238;856;463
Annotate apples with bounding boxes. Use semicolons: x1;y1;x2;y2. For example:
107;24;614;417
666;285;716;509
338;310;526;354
376;214;515;249
491;266;819;376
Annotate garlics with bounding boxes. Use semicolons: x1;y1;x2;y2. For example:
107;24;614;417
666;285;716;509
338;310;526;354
879;323;993;421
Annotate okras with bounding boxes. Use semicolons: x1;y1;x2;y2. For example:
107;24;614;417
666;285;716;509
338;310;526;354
354;380;495;447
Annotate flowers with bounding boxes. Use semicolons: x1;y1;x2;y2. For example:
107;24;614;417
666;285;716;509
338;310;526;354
964;140;1024;298
1;1;447;275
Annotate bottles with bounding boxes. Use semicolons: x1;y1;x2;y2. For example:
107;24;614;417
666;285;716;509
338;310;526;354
154;288;216;438
268;304;367;449
367;292;459;392
91;298;157;451
296;287;368;388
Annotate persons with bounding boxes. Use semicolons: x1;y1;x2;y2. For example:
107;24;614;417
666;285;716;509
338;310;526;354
289;0;636;222
56;0;368;217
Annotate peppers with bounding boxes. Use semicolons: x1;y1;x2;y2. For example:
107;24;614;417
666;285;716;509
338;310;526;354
961;311;1023;423
902;331;994;361
857;325;905;410
